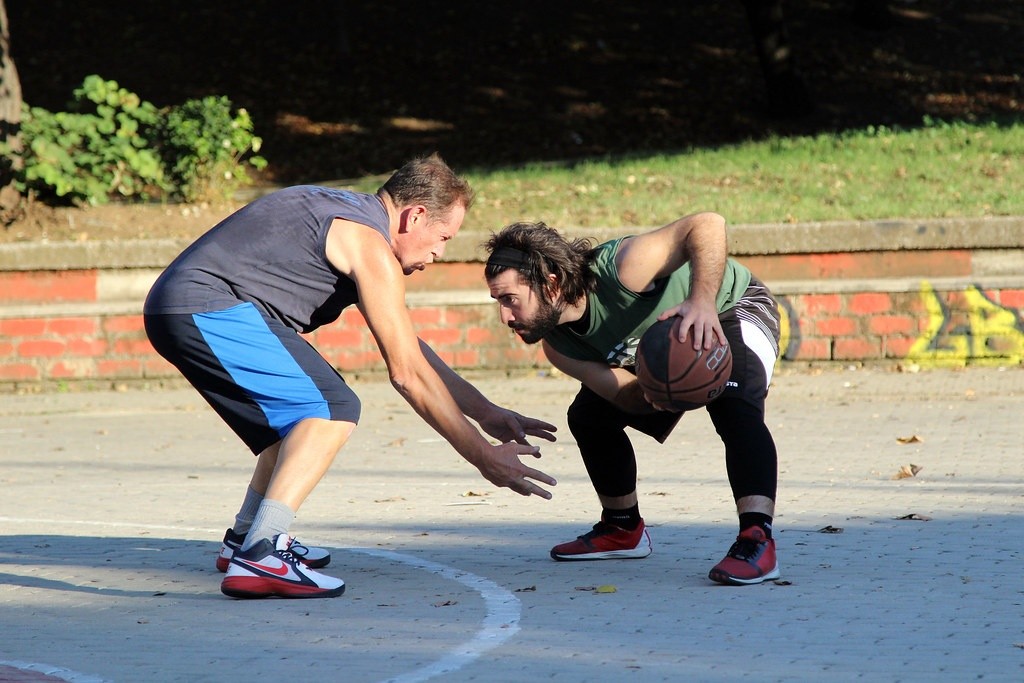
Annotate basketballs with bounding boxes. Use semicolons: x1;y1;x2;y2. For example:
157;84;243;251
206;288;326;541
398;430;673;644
632;314;736;415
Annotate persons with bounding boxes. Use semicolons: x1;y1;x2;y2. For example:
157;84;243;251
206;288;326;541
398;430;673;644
481;210;783;583
141;149;558;600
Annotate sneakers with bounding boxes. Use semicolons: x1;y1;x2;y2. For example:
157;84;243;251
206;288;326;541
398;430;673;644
221;533;345;599
709;525;781;585
216;529;331;573
551;514;653;561
